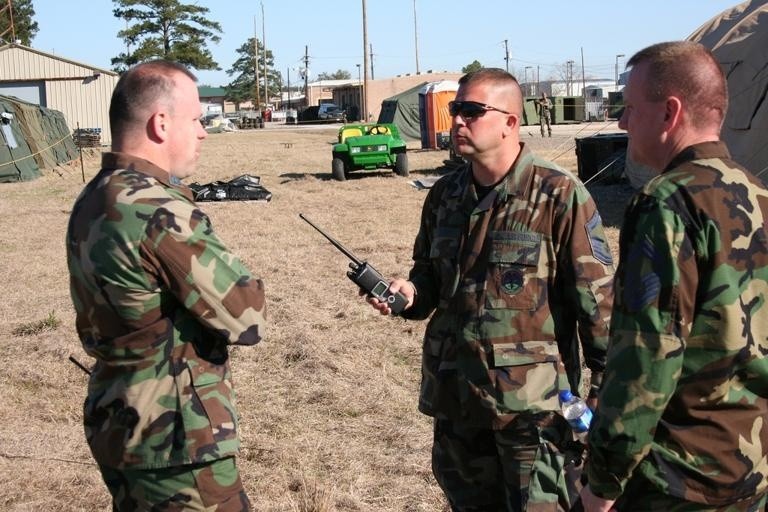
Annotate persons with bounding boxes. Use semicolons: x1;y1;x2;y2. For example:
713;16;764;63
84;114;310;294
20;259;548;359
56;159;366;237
536;91;554;138
66;60;268;512
359;68;615;512
567;40;768;512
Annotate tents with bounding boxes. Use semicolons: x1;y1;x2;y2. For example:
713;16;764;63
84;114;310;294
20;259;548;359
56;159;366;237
0;94;80;183
631;0;768;187
378;80;428;147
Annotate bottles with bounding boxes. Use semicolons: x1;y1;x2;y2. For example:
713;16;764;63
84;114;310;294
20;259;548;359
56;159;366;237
559;389;594;445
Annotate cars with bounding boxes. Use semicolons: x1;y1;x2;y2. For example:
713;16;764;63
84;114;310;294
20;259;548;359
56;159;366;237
200;112;243;129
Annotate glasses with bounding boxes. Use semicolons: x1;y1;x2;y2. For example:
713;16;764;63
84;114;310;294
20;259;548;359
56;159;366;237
449;101;508;118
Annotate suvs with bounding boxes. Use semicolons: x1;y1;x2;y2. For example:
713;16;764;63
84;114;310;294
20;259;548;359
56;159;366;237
318;103;347;123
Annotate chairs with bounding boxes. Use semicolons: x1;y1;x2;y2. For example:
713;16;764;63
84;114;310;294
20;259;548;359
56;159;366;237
340;126;394;145
